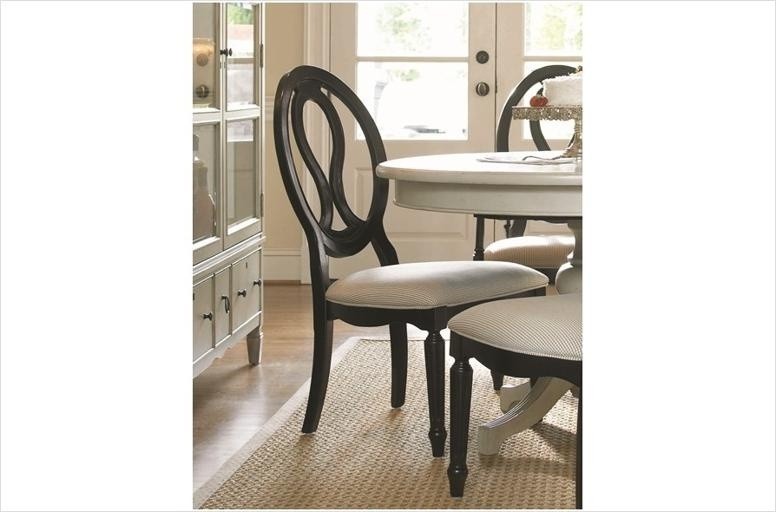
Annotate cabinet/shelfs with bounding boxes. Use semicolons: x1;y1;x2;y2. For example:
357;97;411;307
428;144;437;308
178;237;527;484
194;3;266;269
193;233;267;384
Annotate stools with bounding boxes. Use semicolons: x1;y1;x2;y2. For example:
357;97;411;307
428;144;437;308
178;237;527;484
438;292;582;509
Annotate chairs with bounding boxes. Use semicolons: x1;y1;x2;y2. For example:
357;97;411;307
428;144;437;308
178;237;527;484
273;63;551;462
470;64;581;394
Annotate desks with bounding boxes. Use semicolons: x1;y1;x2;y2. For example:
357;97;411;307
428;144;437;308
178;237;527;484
373;150;581;457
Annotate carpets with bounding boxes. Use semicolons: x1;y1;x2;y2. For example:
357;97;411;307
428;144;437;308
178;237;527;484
195;332;580;507
